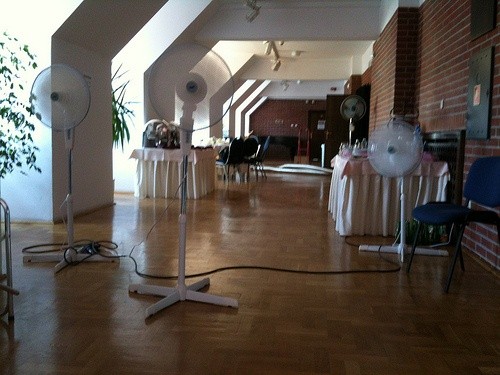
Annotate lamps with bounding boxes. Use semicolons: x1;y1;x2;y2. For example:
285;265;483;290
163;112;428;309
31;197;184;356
282;84;289;91
272;57;280;71
265;43;272;55
246;8;259;23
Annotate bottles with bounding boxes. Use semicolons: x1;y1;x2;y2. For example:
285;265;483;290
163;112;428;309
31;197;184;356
361;138;368;151
354;139;360;149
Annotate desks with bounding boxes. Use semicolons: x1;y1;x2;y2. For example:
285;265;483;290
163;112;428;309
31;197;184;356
129;148;217;199
328;154;450;236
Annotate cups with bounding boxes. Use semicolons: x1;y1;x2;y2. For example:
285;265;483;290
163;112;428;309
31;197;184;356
352;147;361;158
339;141;353;155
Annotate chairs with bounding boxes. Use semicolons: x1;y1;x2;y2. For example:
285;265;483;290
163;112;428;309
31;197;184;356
216;135;270;190
407;156;500;291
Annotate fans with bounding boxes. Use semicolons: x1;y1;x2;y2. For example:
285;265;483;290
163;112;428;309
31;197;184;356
128;44;238;317
340;95;366;148
359;121;448;261
23;64;120;272
